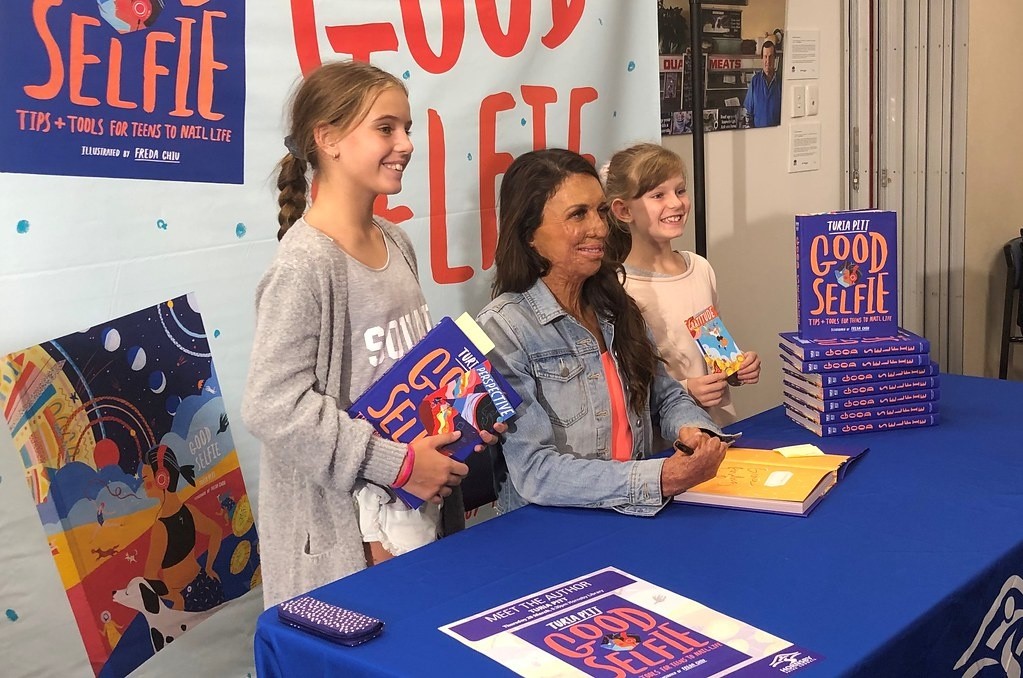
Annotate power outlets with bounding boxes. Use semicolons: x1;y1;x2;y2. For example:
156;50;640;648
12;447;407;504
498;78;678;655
806;85;819;116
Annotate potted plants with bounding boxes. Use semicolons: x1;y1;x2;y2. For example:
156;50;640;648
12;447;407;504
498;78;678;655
658;6;689;54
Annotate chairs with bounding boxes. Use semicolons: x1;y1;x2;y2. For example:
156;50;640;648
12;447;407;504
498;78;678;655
998;237;1023;380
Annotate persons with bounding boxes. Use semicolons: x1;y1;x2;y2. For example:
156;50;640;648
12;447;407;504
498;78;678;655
240;67;508;613
597;141;761;454
473;147;744;518
744;41;781;127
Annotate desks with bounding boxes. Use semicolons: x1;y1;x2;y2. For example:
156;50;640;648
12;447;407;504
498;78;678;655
255;370;1023;678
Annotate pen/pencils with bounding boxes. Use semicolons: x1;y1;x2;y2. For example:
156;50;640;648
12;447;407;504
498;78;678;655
675;441;694;455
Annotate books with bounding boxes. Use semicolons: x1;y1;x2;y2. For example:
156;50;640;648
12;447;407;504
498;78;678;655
683;304;746;382
345;316;523;509
673;437;871;518
778;208;941;436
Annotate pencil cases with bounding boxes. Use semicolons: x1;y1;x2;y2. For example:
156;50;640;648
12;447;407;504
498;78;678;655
278;597;385;646
